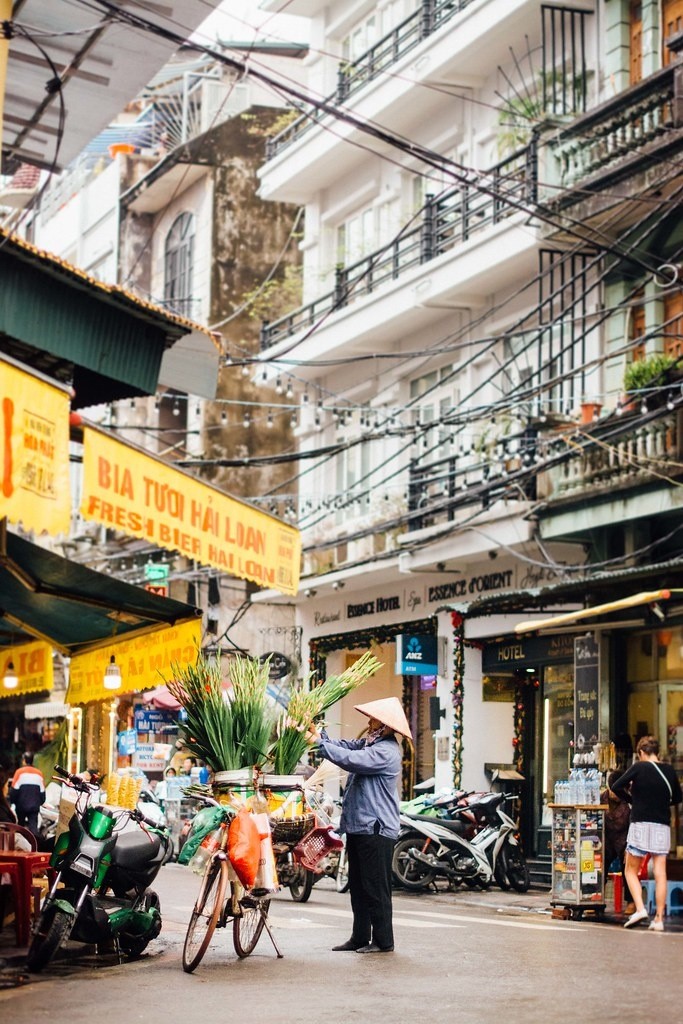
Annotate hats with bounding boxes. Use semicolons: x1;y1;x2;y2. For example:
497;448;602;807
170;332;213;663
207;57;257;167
352;696;414;741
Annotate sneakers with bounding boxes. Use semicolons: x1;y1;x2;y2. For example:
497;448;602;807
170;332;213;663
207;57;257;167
622;909;648;928
648;921;667;933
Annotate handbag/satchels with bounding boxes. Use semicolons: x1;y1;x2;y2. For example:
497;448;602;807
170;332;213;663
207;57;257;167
227;810;279;892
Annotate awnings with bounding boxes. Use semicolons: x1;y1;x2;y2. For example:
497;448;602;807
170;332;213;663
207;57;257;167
0;528;203;705
0;227;225;415
428;558;683;616
2;0;226;163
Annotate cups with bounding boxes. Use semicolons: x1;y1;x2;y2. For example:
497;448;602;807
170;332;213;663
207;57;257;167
572;751;599;765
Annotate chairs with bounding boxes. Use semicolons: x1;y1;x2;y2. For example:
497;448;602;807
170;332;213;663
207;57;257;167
0;822;56;947
609;852;651;912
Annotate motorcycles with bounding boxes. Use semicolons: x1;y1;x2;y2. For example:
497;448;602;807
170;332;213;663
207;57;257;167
25;763;175;974
272;843;317;903
313;769;531;894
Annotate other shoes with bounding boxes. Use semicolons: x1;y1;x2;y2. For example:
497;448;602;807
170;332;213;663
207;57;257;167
331;938;396;953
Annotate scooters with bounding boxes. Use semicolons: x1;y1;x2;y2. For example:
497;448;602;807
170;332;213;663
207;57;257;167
135;787;173;865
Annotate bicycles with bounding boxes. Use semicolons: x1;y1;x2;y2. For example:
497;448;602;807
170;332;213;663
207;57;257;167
182;791;284;972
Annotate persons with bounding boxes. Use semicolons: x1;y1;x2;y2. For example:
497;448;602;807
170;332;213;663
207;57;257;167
600;736;683;932
6;751;46;834
292;696;413;953
164;739;214;783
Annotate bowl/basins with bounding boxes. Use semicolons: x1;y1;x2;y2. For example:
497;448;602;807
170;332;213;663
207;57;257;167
108;143;136;158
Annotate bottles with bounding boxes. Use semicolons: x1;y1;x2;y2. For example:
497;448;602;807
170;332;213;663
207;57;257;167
568;768;600;805
554;781;571;805
106;773;143;809
136;744;172;768
166;775;191;799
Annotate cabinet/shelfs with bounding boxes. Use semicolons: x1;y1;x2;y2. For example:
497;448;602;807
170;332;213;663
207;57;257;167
548;803;609;919
165;799;195;855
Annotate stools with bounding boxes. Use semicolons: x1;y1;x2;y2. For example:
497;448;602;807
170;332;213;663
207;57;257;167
640;880;655;915
667;882;683;915
295;825;344;873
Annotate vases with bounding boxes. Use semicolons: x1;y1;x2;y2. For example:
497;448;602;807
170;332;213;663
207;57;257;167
624;357;683;413
580;403;603;426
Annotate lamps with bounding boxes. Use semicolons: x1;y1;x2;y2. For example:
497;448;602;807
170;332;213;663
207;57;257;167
4;662;18;688
104;655;120;689
